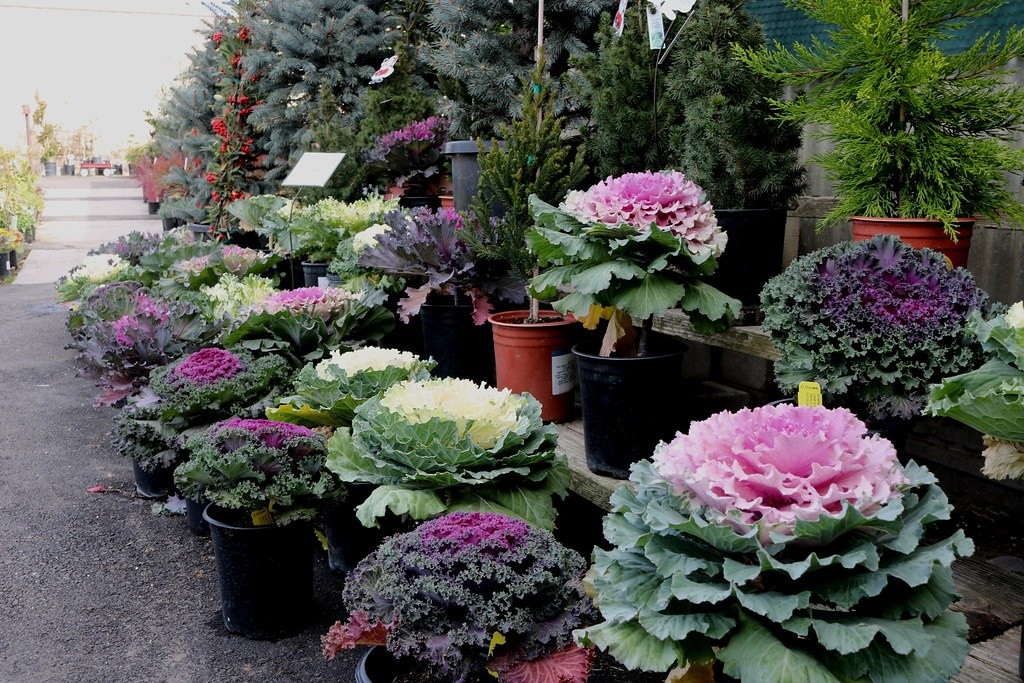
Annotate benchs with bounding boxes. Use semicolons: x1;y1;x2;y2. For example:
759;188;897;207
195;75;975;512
547;307;1024;683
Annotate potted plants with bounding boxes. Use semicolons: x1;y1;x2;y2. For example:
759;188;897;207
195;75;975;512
736;0;1024;276
456;68;585;423
427;0;542;210
656;0;807;326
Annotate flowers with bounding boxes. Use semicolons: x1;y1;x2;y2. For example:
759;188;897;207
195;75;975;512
57;226;1024;683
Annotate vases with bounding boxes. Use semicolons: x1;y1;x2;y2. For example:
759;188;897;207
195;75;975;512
571;342;696;479
189;223;211;244
188;413;232;432
326;272;345;287
202;502;320;637
354;648;470;683
301;263;330;288
415;297;496;379
